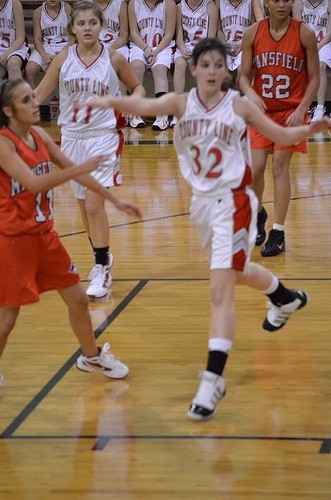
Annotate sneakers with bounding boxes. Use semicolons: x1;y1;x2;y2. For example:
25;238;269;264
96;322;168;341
255;206;268;246
85;254;113;298
260;230;285;256
170;115;178;128
77;346;128;378
122;112;146;127
263;290;307;332
189;372;226;420
310;105;326;122
151;113;169;130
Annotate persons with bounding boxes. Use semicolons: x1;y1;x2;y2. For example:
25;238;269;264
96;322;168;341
77;37;331;421
0;79;142;378
26;0;76;87
126;0;177;130
240;0;320;257
170;0;218;126
293;0;331;123
216;0;270;89
88;0;129;96
33;0;145;298
0;0;31;80
